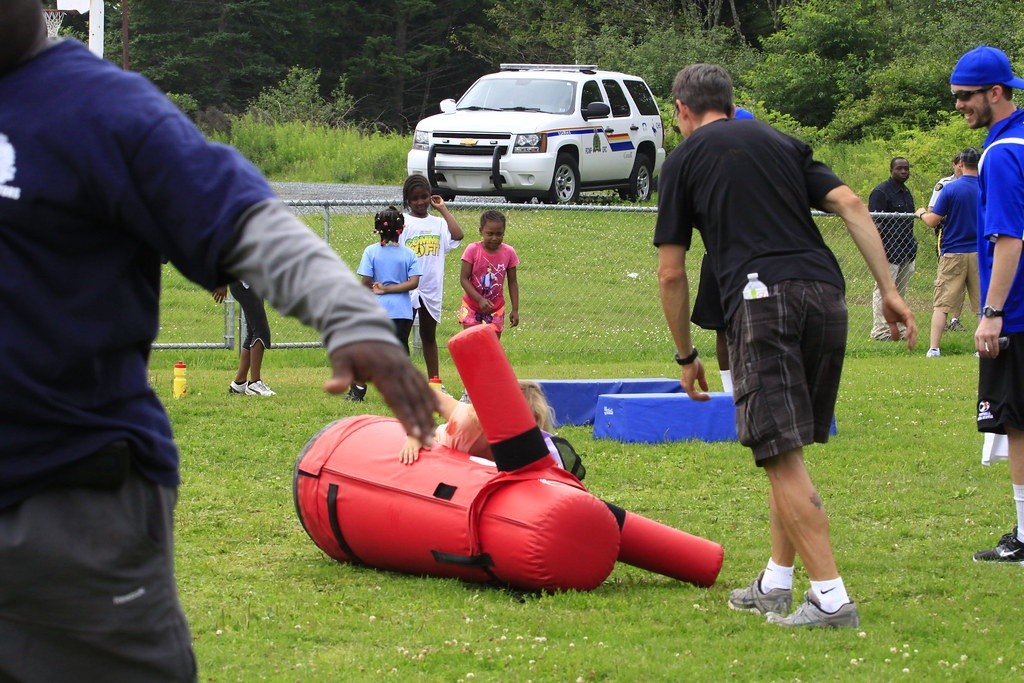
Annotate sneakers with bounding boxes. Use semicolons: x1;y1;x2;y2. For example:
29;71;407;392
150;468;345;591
972;525;1023;563
245;379;277;396
727;569;793;615
949;320;964;331
229;376;249;393
926;347;940;357
766;589;860;628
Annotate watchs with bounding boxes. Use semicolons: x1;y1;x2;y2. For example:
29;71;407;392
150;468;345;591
982;306;1003;318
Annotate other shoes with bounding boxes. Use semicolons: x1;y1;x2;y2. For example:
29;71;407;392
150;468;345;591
344;384;366;403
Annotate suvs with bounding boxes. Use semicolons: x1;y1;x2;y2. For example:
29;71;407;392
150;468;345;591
407;62;667;205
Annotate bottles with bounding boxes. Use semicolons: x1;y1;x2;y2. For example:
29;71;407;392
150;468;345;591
173;361;187;400
742;273;769;299
428;376;442;418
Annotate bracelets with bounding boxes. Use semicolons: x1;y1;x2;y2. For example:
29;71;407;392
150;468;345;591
675;345;698;365
920;212;926;218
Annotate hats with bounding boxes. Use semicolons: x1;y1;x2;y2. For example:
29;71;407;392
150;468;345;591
950;45;1024;90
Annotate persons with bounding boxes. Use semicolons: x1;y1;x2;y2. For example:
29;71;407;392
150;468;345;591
734;106;753;120
459;210;520;340
868;157;918;341
212;280;277;396
653;64;917;629
398;174;464;398
434;379;557;462
344;206;423;403
914;147;981;357
928;154;967;332
0;0;439;683
950;44;1024;565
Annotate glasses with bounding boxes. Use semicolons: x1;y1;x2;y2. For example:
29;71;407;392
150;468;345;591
999;337;1011;350
954;86;993;101
671;99;686;133
